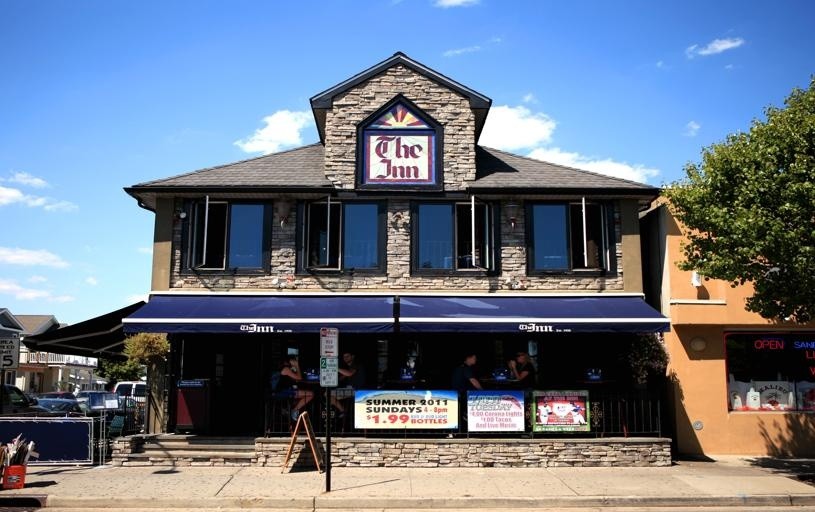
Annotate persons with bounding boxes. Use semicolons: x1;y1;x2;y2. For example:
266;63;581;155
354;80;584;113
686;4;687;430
324;348;365;417
451;344;484;426
275;351;316;421
508;346;537;401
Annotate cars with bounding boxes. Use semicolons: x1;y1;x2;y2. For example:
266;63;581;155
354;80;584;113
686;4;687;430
0;384;120;417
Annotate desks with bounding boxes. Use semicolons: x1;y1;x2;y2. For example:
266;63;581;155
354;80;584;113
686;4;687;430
302;380;321;432
576;380;608;400
480;378;512;390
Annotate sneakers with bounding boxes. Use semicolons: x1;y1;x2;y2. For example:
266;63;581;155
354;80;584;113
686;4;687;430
338;411;348;419
290;408;300;422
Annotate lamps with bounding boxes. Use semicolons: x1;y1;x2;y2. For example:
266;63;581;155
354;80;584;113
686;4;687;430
173;209;186;220
274;195;294;230
690;338;707;352
504;197;521;231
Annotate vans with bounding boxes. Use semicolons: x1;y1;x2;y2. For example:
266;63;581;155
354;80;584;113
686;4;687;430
112;381;146;402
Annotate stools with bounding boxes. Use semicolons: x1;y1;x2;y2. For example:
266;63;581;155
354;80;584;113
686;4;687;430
268;396;291;431
